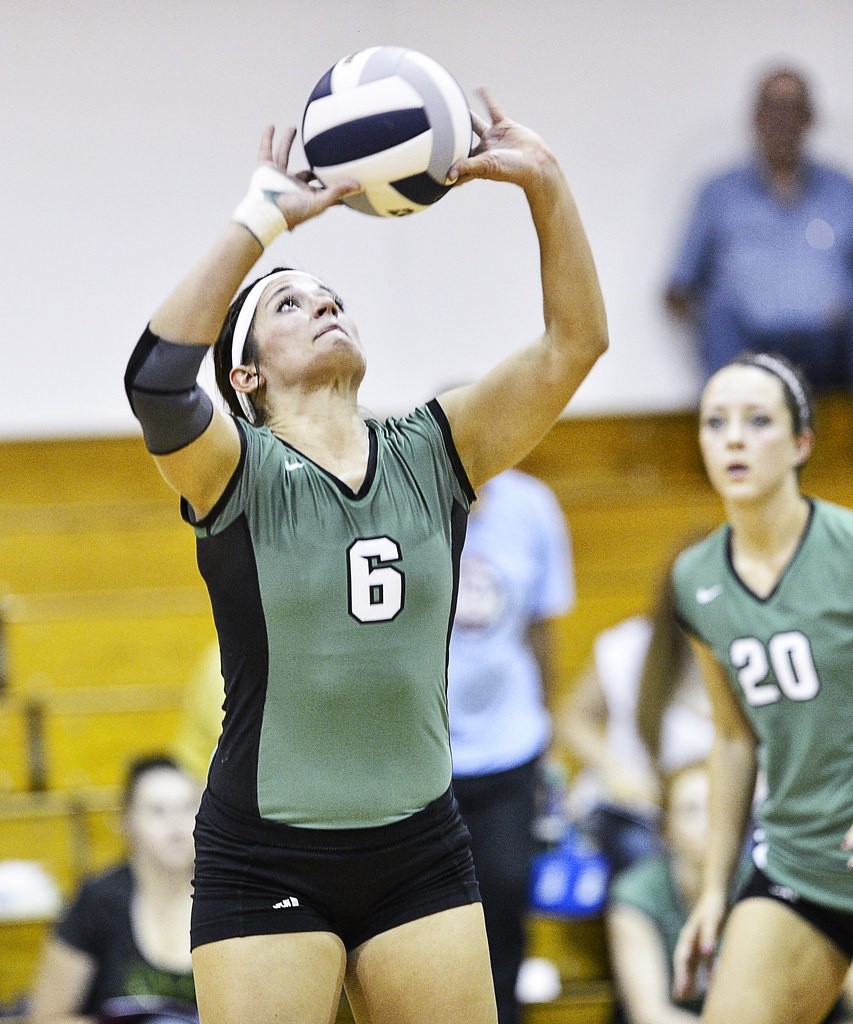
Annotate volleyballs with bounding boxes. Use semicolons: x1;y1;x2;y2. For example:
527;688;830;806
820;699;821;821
299;43;475;222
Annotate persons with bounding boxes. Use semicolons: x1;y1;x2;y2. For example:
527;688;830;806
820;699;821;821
555;70;853;1024
26;88;608;1024
